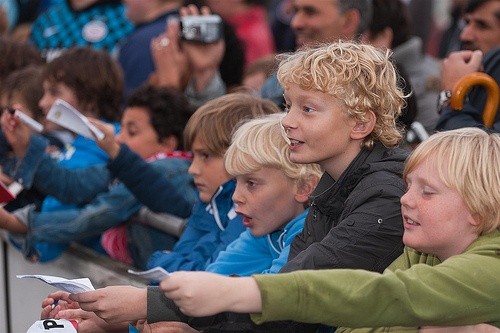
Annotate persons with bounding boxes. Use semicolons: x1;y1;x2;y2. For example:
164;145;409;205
148;92;283;285
437;0;500;136
69;41;411;333
41;111;325;333
0;0;442;272
160;127;500;333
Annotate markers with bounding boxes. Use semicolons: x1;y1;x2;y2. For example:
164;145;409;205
9;108;65;149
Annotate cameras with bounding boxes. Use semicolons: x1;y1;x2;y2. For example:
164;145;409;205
180;15;222;44
403;121;428;147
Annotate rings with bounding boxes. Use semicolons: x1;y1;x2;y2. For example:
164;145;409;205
158;37;170;47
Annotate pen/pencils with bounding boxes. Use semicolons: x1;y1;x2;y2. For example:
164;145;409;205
51;299;59;309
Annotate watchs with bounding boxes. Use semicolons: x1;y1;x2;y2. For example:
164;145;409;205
437;89;453;112
